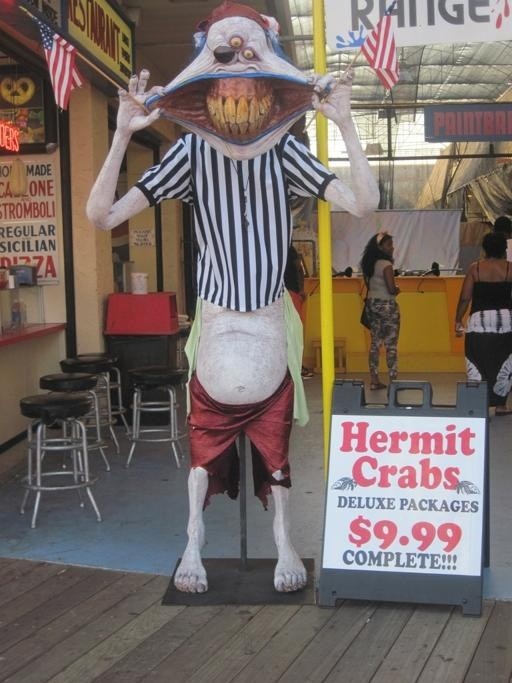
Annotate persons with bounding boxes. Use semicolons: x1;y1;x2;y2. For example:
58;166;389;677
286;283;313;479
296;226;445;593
492;214;511;261
284;242;315;378
453;233;511;414
361;230;402;390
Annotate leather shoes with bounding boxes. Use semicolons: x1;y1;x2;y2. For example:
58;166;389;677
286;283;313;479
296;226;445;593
369;382;386;390
495;407;511;416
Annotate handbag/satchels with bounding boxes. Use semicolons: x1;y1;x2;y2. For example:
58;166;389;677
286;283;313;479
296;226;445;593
360;302;371;331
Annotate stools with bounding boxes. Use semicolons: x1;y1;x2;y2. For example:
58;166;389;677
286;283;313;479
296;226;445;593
310;336;347;375
20;392;103;528
39;371;111;472
76;352;132;439
58;354;120;456
126;365;189;468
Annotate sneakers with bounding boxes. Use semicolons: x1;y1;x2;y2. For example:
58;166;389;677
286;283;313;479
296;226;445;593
300;366;314;379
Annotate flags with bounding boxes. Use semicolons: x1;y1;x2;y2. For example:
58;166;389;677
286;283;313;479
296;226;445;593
360;10;401;91
37;17;85;113
